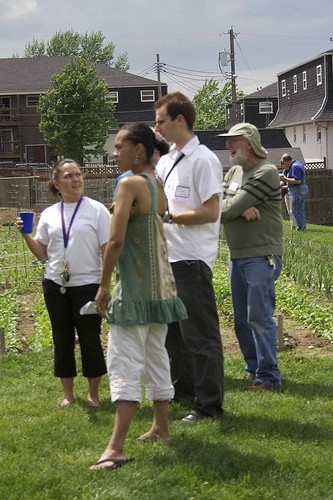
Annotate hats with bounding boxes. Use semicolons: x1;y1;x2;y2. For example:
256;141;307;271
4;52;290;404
215;123;269;158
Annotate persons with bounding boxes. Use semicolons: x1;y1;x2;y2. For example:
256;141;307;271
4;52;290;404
153;92;225;423
89;122;188;471
218;123;282;392
277;154;310;231
17;160;113;408
112;126;171;203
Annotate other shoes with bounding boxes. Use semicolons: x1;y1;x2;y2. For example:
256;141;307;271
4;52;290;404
179;410;213;423
233;375;281;394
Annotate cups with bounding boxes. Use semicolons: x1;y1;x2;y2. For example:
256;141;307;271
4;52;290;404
20;212;34;233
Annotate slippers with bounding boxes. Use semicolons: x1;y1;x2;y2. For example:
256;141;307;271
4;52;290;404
87;458;128;471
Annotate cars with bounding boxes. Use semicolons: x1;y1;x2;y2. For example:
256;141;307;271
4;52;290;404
15;162;48;168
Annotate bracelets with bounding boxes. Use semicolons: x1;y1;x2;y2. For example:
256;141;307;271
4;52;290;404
169;214;173;224
